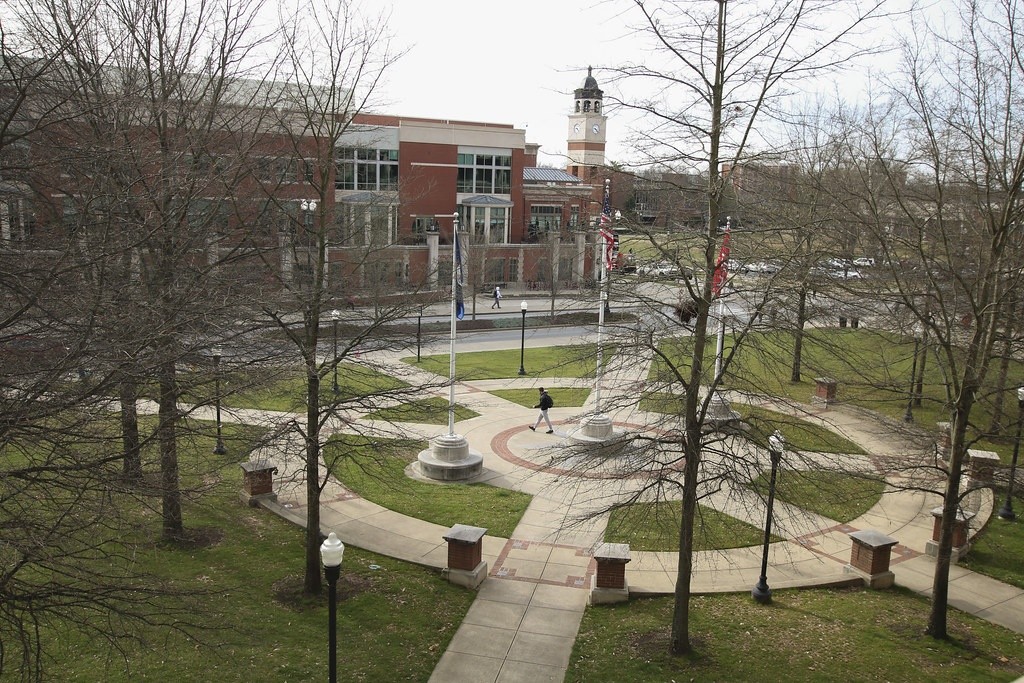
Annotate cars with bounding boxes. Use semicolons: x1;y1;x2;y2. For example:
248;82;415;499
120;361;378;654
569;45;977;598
618;252;906;283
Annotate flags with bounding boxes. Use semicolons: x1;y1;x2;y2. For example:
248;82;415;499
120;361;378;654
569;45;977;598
713;229;730;299
599;189;624;272
455;229;464;320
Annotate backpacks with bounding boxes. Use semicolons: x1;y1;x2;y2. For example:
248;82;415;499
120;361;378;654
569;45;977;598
493;290;497;298
544;393;553;408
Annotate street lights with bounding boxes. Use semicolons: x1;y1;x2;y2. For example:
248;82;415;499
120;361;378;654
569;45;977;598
301;198;318;289
903;325;922;424
751;430;785;605
1000;385;1024;521
211;345;228;455
321;532;345;683
717;306;728;385
331;308;341;393
414;303;422;364
516;300;529;376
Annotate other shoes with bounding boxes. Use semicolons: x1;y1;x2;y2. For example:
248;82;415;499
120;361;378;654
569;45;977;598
546;430;553;433
529;426;535;431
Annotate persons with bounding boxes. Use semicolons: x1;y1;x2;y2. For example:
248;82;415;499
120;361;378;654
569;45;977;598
492;287;502;309
533;284;536;290
529;387;554;434
